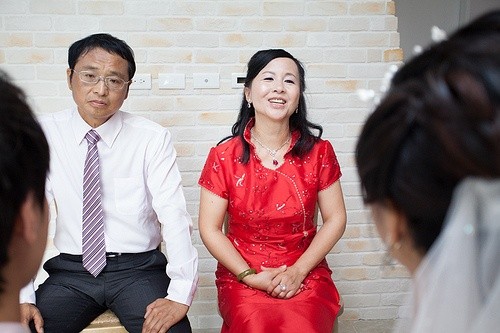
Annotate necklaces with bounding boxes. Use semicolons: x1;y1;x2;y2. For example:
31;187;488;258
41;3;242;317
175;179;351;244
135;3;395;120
248;128;291;166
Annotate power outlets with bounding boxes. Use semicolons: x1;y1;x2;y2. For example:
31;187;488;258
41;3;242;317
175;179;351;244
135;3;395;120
129;74;152;90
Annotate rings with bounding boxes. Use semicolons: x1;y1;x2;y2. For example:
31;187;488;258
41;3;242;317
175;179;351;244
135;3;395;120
280;283;287;291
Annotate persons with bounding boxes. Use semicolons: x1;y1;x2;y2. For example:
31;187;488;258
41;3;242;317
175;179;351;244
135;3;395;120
354;7;500;333
197;49;347;333
0;71;50;333
19;33;198;333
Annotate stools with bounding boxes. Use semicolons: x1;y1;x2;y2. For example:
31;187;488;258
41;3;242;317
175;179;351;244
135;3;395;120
79;309;132;333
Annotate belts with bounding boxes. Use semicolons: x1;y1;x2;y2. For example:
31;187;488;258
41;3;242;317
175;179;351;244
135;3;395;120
106;252;122;257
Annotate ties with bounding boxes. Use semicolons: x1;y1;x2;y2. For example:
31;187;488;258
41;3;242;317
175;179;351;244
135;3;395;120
82;131;107;277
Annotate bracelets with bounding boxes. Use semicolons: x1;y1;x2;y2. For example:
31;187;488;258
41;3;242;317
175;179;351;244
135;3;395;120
237;269;256;282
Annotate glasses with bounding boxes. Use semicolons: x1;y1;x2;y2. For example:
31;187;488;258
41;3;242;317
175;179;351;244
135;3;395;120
73;70;130;90
